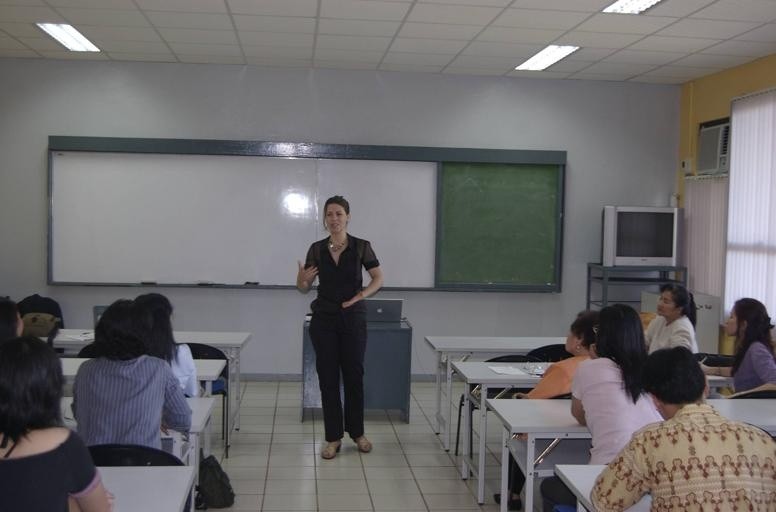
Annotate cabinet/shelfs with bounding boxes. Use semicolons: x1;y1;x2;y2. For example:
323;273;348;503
586;262;688;313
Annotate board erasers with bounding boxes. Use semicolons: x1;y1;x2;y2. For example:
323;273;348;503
245;281;260;286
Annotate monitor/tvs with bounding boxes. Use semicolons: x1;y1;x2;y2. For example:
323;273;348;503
602;206;685;267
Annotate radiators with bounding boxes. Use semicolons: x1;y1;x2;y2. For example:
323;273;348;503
680;175;729;298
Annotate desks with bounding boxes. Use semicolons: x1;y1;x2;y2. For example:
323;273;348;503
36;329;252;512
426;336;776;512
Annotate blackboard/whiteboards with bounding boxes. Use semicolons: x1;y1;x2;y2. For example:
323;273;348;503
47;136;567;294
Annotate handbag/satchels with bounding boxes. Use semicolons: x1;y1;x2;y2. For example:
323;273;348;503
22;312;60;351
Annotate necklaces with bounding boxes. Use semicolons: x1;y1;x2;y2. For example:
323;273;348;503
329;240;345;252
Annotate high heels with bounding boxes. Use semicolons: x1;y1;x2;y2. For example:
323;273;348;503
322;438;341;460
355;435;373;453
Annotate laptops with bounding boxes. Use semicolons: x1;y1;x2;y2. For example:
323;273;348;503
366;298;403;322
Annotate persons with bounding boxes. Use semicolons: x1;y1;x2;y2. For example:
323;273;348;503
643;284;698;355
134;293;201;398
589;346;776;512
296;196;384;459
493;311;600;512
540;303;664;512
0;298;24;344
0;335;115;512
698;297;776;399
70;299;193;451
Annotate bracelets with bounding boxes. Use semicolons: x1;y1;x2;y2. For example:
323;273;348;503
719;367;721;376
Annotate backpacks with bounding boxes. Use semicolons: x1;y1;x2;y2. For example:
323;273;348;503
197;447;236;510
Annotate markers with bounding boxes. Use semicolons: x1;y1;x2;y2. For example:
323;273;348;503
140;282;156;285
